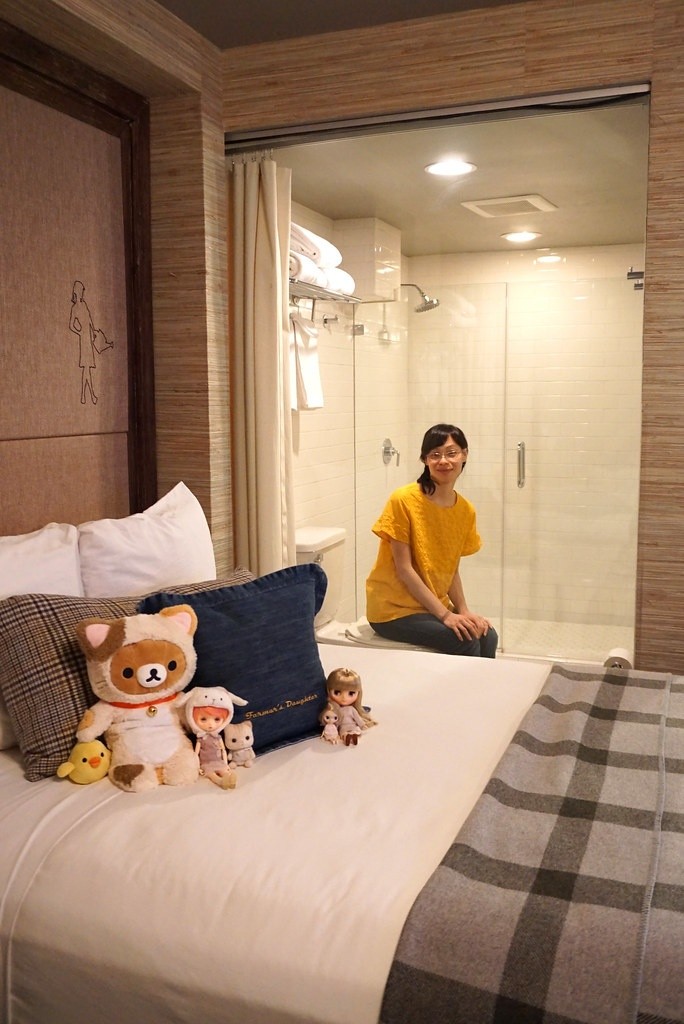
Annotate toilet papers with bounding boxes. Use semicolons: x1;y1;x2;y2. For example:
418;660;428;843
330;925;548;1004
602;648;634;669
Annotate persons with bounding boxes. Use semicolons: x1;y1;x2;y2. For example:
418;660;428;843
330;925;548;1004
172;686;248;790
326;668;378;746
365;423;498;659
321;707;342;744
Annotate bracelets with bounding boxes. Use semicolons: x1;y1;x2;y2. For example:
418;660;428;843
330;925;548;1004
440;610;452;624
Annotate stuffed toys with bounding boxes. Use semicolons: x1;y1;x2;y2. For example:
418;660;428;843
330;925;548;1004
224;722;256;770
57;740;112;785
74;604;202;792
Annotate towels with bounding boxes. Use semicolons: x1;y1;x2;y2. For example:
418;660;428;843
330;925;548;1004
290;221;356;298
289;313;324;411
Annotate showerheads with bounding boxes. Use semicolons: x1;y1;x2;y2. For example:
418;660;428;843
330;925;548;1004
400;284;440;313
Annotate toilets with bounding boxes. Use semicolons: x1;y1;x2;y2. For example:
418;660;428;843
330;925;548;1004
295;526;453;653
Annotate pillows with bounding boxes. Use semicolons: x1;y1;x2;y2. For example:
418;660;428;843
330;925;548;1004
77;480;217;599
138;562;371;764
0;522;86;751
0;570;258;784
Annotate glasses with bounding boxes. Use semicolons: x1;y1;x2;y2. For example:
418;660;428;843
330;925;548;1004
425;450;462;461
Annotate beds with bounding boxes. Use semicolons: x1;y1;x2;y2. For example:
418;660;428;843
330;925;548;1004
0;641;684;1024
